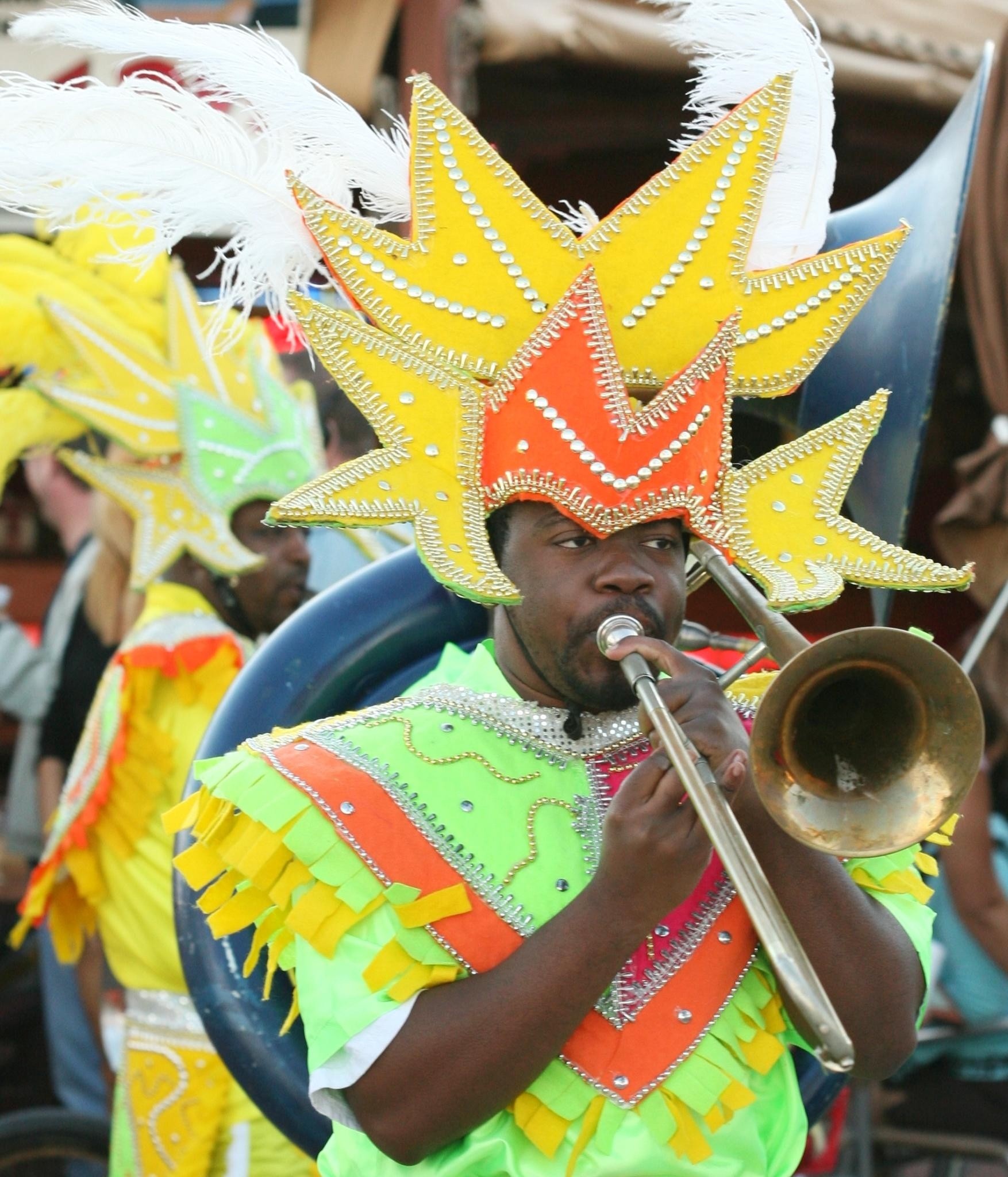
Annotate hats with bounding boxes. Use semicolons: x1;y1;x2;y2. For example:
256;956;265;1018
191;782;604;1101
0;0;977;616
0;184;413;591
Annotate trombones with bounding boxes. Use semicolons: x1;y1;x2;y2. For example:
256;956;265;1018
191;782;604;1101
595;538;985;1072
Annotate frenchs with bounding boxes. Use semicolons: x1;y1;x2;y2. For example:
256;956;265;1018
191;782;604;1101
172;36;997;1165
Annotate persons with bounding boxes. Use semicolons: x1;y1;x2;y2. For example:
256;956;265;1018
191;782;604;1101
0;257;329;1177
161;90;977;1177
911;729;1007;1134
